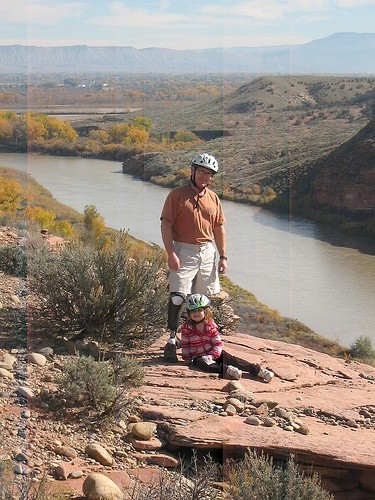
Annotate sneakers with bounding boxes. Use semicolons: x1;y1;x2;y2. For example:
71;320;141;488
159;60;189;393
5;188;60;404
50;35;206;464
226;366;242;380
257;368;273;380
164;343;178;362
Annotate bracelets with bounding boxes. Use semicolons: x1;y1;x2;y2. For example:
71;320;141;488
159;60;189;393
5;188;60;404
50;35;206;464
220;256;227;261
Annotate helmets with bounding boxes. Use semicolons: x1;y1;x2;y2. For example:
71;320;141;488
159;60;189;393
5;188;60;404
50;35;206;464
186;293;210;310
192;153;218;172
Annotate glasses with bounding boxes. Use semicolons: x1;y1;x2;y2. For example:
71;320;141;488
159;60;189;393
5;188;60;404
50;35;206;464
198;169;211;176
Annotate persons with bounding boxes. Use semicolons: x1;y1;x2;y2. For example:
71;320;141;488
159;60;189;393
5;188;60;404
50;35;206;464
160;154;228;363
180;293;274;383
